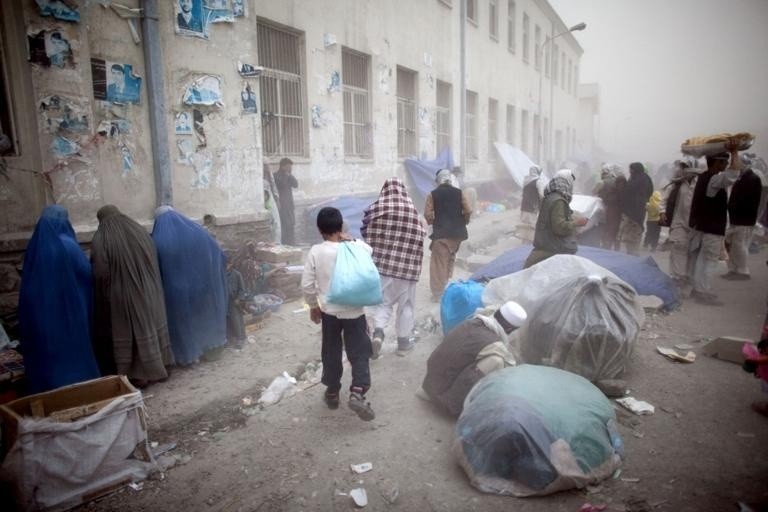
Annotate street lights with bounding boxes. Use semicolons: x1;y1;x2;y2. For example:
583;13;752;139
535;19;587;169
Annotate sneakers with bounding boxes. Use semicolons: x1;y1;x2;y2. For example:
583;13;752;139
397;337;415;357
347;392;376;422
689;288;723;306
721;271;750;281
324;388;340;410
369;330;385;361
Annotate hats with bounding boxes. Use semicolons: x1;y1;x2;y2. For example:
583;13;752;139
499;300;528;328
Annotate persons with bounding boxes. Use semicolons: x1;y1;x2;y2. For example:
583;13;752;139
667;137;768;307
222;251;247;350
300;206;375;421
519;159;664;270
148;205;229;366
272;157;298;247
422;302;527;417
90;205;175;388
20;205;103;397
424;168;472;303
360;179;427;359
